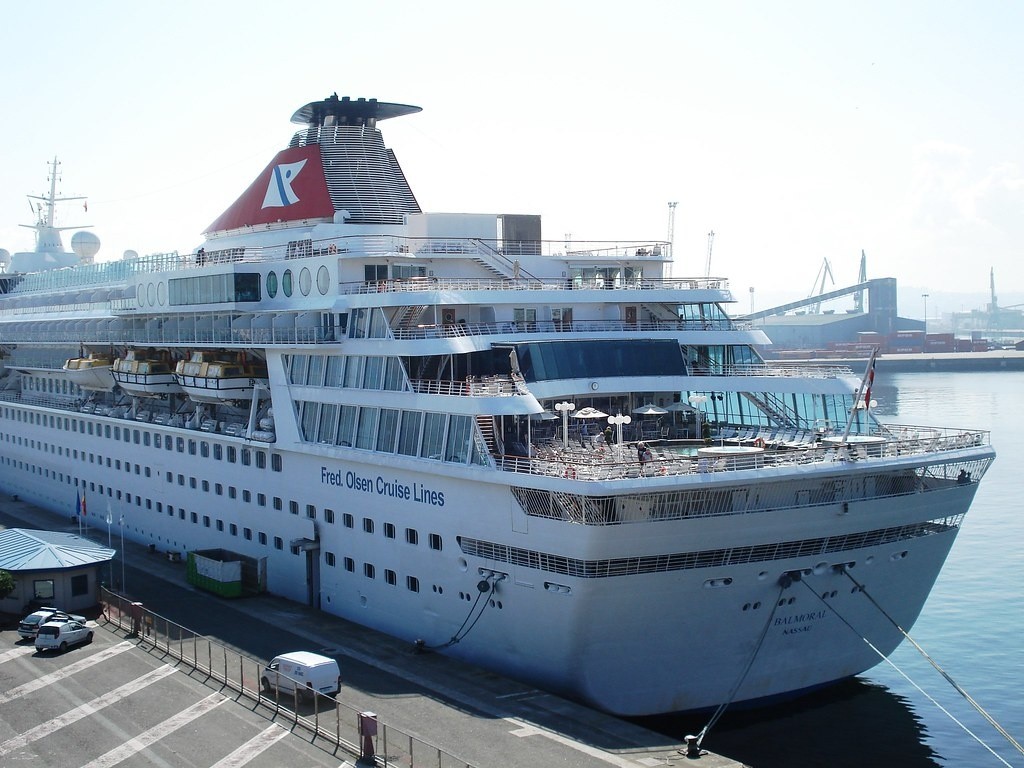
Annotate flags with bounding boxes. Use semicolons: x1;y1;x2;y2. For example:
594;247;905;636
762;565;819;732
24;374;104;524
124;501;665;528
865;358;875;410
76;488;124;526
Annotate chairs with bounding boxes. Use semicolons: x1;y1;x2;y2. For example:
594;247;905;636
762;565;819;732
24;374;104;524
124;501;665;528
473;377;512;397
711;427;982;462
512;427;727;482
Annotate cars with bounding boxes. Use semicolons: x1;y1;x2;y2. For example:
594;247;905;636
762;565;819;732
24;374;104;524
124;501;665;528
34;619;95;652
17;606;87;639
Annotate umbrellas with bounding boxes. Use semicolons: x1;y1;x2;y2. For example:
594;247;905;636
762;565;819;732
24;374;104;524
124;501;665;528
523;402;695;428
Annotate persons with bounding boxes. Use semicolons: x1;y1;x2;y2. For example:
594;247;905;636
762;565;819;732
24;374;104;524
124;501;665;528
637;442;653;465
604;426;612;445
195;247;206;267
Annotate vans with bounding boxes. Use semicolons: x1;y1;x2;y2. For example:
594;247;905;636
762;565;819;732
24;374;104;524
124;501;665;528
260;650;343;705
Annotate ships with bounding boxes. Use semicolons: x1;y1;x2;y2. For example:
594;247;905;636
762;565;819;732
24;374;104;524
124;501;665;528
0;92;997;717
